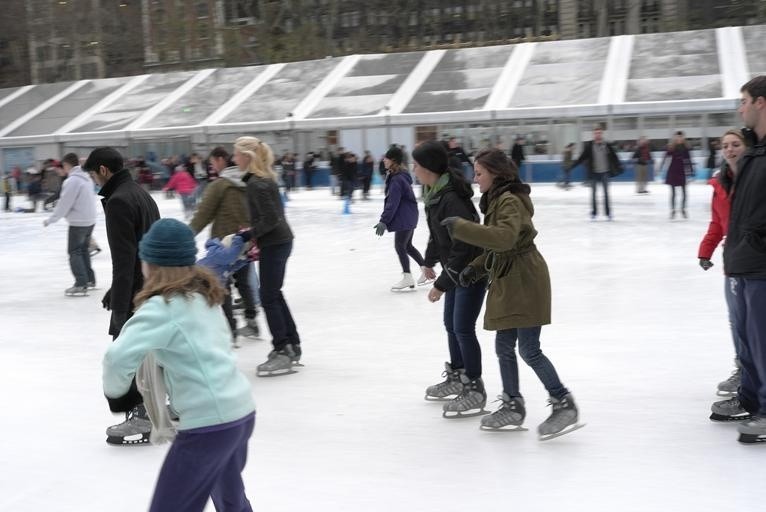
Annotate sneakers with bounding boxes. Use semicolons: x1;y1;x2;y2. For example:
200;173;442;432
718;358;741;392
86;280;95;287
426;362;465;396
106;409;152;437
738;415;766;435
257;351;292;371
443;374;486;410
539;392;577;435
65;285;87;293
268;345;300;361
392;272;414;289
482;392;525;426
88;240;97;251
712;396;749;416
418;264;431;281
234;318;258;336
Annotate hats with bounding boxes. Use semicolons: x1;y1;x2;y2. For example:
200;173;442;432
220;233;248;260
138;219;197;267
386;147;403;164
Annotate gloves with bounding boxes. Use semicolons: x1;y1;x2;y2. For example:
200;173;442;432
239;230;252;242
374;223;386;235
441;217;456;239
103;287;111;310
699;258;713;270
112;310;127;334
460;265;475;286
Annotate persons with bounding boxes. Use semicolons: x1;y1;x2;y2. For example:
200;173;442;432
696;128;760;424
708;74;765;444
439;147;586;442
0;118;724;220
42;151;103;298
81;147;161;445
195;224;259;288
411;137;492;419
230;135;305;378
185;144;260;346
372;145;436;294
100;218;257;511
